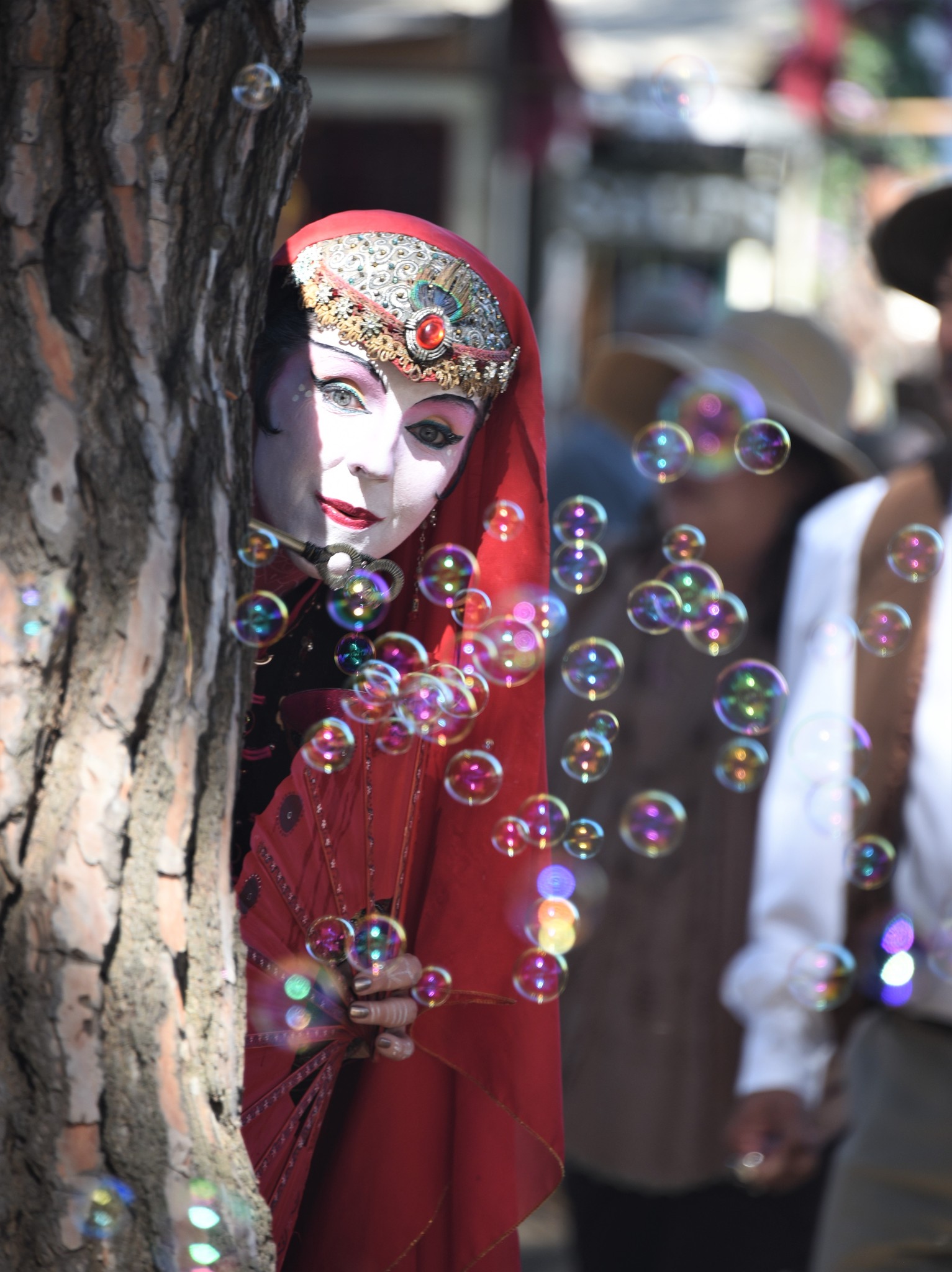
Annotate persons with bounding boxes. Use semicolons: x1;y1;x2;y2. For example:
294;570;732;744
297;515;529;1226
545;310;879;1272
231;209;566;1272
717;181;951;1272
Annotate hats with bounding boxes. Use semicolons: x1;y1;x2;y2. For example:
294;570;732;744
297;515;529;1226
618;309;878;482
872;188;952;302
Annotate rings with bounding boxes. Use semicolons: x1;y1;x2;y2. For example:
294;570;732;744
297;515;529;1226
734;1154;763;1179
